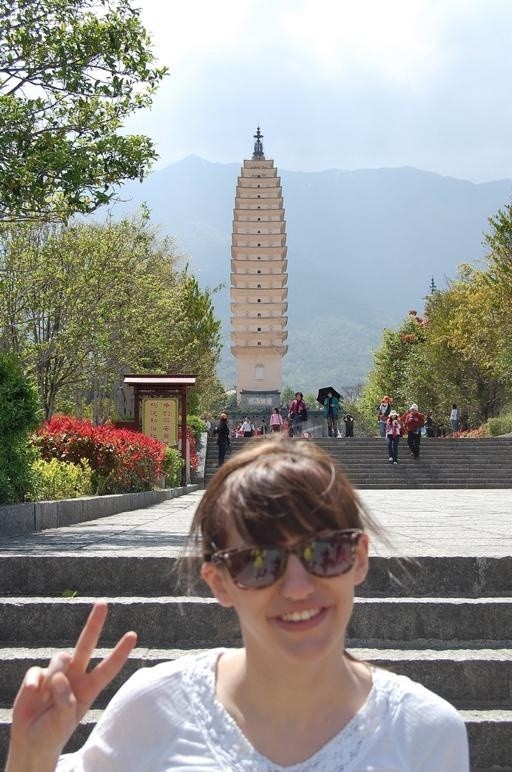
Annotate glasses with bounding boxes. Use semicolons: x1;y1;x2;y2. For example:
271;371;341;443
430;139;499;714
200;527;363;591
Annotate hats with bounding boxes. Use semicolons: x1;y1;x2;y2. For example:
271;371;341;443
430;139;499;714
380;396;419;417
219;412;227;420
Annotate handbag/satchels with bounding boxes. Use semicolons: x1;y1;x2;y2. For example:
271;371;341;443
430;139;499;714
288;409;307;422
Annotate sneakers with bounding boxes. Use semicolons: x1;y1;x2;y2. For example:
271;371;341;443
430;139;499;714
387;452;420;465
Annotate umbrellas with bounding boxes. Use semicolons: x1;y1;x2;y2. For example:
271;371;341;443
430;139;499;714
317;387;341;405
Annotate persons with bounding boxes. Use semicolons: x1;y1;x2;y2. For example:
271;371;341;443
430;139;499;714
5;435;470;771
215;392;458;466
